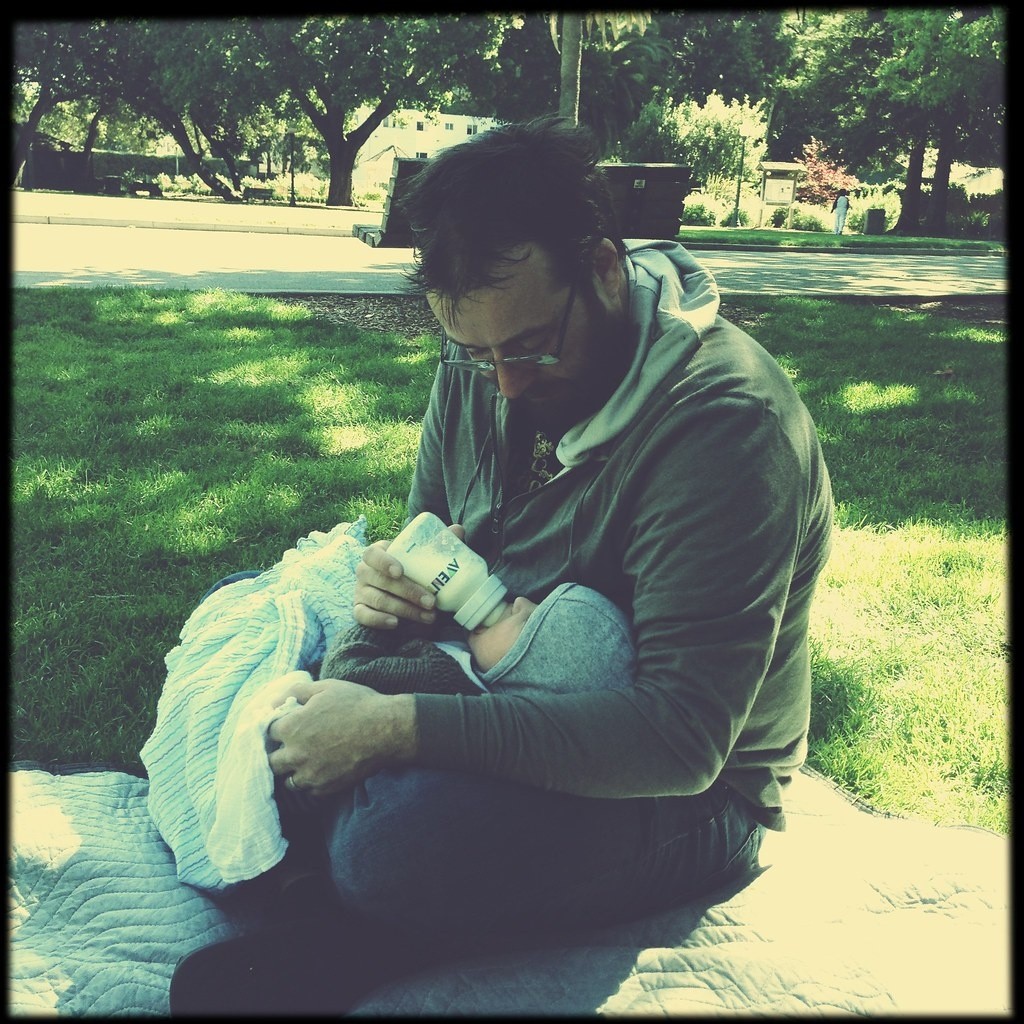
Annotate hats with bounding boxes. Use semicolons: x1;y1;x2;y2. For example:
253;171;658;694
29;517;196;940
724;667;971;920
473;581;639;696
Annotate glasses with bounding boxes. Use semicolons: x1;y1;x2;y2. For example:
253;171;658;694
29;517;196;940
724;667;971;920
440;252;587;373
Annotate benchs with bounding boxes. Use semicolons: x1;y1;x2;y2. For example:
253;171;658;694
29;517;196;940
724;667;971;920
240;186;274;204
131;182;162;195
354;156;694;249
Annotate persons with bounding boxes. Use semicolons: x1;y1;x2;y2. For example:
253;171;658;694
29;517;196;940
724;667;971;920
165;114;834;1016
318;582;638;695
829;188;850;233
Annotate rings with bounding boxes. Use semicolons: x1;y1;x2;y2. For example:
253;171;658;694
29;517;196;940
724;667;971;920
289;777;303;792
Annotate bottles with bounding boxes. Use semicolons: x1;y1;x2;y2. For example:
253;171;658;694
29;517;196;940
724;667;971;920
384;511;509;631
